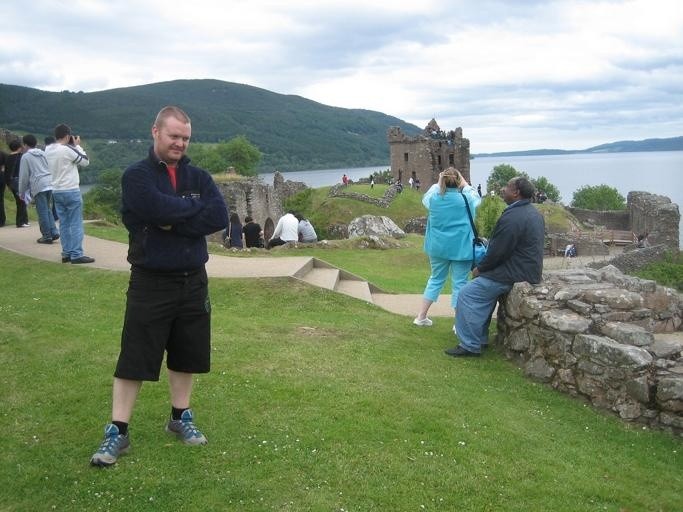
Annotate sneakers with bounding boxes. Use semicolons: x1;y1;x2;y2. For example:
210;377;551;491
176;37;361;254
70;256;94;264
413;317;433;327
445;339;490;358
37;237;52;244
18;222;31;227
88;424;131;467
52;235;59;241
165;409;208;448
61;256;69;264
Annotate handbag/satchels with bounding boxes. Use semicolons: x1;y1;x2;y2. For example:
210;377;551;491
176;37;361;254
9;176;18;191
472;236;489;268
223;236;230;249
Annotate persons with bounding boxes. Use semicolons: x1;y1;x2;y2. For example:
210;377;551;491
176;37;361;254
564;244;575;258
412;166;482;336
298;217;318;243
443;176;546;357
0;122;96;264
87;105;230;468
268;209;299;247
242;215;265;248
226;212;244;250
342;174;348;188
369;126;547;204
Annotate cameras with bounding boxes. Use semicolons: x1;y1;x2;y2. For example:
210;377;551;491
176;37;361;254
69;136;77;143
